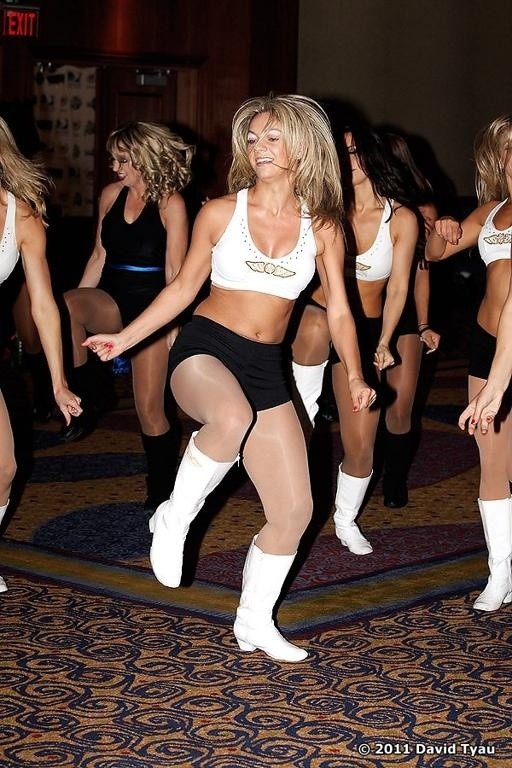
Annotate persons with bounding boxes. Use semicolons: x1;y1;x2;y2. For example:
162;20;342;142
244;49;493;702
290;112;418;555
457;276;512;435
61;120;198;510
0;116;82;594
425;115;512;612
367;122;441;507
81;94;379;663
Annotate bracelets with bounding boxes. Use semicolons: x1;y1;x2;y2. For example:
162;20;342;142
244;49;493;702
419;326;429;337
417;322;428;328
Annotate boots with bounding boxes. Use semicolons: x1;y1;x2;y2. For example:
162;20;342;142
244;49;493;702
63;360;98;442
292;360;329;427
0;499;11;593
233;532;308;662
149;430;241;589
383;422;411;510
333;462;374;556
140;417;180;518
472;496;512;612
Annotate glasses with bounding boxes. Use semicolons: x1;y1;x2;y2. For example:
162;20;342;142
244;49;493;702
348;144;358;155
